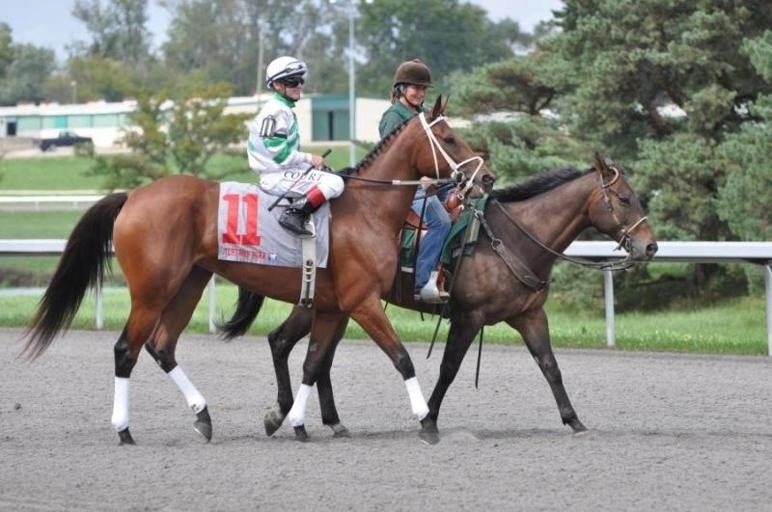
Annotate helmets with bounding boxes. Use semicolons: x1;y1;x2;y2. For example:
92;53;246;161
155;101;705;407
394;58;434;88
266;56;307;90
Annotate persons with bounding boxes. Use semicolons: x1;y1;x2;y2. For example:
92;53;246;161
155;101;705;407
378;59;453;302
247;55;346;235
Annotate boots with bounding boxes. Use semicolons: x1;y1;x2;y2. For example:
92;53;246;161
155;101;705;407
279;186;326;235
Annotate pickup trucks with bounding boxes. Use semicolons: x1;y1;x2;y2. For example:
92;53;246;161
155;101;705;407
35;130;94;151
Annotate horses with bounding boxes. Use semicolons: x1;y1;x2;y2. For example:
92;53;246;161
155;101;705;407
15;92;499;445
210;150;659;438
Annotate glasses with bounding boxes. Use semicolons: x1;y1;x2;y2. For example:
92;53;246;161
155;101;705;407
279;77;304;87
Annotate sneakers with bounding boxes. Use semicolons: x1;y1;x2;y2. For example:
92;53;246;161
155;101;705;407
413;287;450;302
